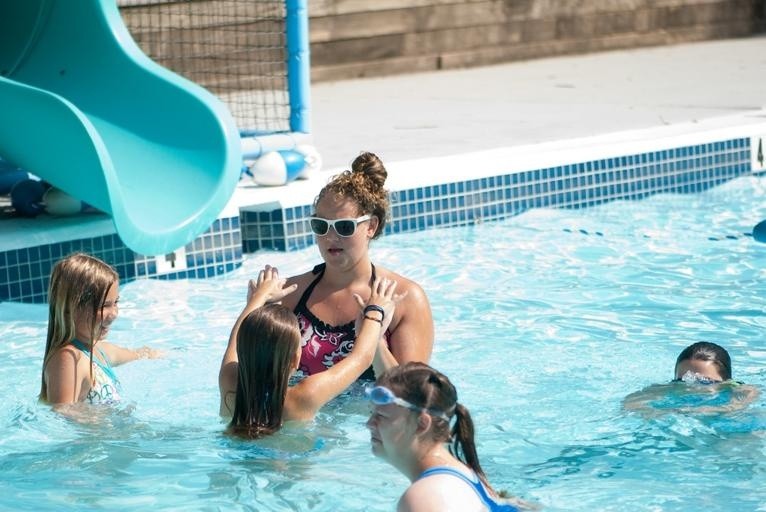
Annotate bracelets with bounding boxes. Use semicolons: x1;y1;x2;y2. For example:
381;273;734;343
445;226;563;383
361;314;383;327
362;304;385;321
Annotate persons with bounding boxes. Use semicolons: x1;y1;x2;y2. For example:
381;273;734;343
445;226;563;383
36;253;164;433
247;150;431;413
622;340;757;421
216;264;408;441
365;362;534;512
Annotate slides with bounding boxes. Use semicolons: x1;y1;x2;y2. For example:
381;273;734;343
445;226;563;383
0;1;243;258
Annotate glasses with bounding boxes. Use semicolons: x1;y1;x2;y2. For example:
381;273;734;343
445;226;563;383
672;377;722;385
309;214;370;238
361;385;450;422
